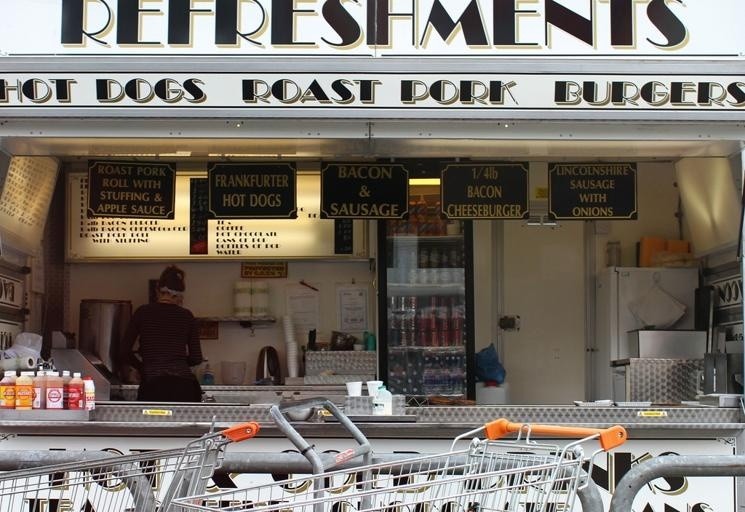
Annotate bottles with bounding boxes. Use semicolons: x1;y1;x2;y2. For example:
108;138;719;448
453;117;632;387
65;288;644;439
388;353;468;394
372;385;393;414
0;371;96;412
420;246;460;268
606;240;621;267
198;355;215;384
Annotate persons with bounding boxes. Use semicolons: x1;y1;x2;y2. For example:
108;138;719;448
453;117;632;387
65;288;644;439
121;266;203;402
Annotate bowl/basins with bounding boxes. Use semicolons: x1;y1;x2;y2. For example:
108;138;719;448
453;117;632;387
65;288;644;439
287;407;314;420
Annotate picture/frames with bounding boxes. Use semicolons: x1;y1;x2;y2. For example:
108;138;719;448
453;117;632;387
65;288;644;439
0;155;63;258
64;171;371;264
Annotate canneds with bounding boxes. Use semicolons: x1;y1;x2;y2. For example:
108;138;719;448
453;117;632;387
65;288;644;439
386;295;464;347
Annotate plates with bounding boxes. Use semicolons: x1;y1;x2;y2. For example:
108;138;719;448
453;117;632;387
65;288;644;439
307;328;376;350
573;399;652;407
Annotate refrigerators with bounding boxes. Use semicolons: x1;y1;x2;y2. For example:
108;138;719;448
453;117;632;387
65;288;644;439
377;186;475;407
595;266;701;401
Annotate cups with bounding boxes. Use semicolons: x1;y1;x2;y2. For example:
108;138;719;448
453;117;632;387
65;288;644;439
282;315;301;376
343;396;406;415
221;361;247;385
367;380;384;395
447;223;462;235
345;381;363;396
